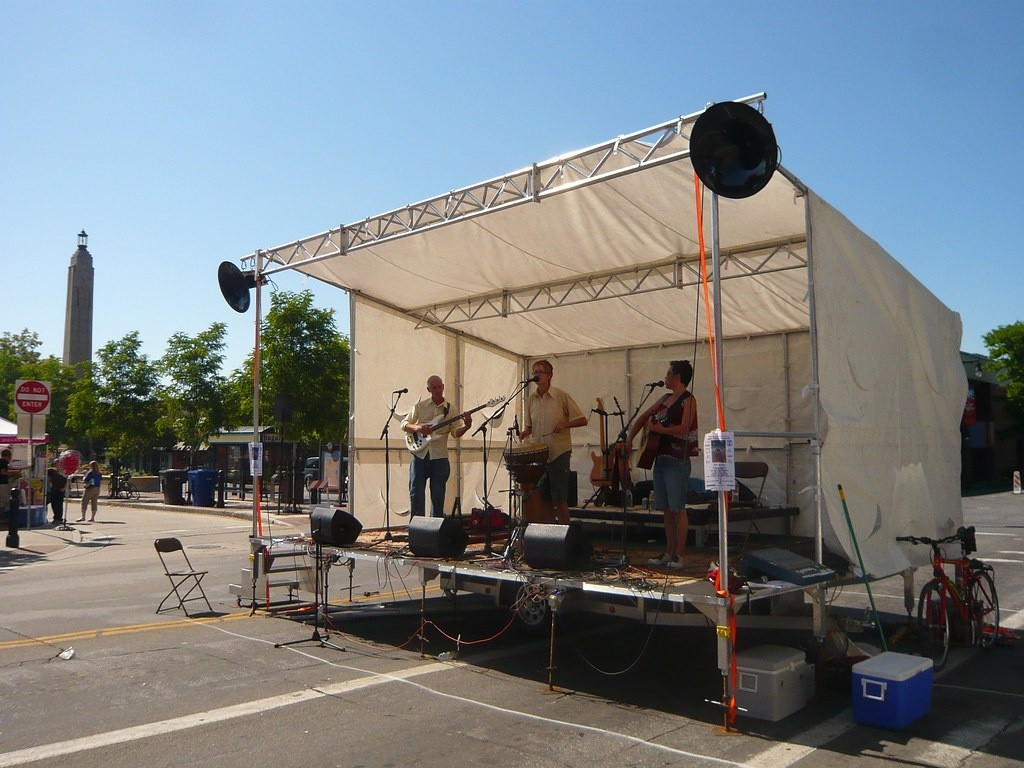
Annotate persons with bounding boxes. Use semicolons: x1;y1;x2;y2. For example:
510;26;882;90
0;449;20;510
79;460;103;521
401;376;473;523
520;360;588;526
625;360;697;569
47;459;68;525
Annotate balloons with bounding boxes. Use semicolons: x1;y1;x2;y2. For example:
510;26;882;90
58;449;82;478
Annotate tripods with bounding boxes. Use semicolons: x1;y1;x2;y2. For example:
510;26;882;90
582;408;626;510
469;425;522;565
274;531;346;651
365;392;409;549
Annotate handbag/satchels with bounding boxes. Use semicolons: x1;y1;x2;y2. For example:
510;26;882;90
84;472;94;489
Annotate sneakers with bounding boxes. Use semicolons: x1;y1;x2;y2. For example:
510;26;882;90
648;553;670;565
668;554;684;569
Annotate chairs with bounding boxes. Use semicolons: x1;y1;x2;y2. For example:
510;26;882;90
154;538;213;616
706;461;769;558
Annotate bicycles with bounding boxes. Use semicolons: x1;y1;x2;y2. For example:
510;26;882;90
895;525;1000;672
107;473;140;500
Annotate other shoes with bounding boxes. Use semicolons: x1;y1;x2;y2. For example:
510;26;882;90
88;518;94;521
52;519;63;524
76;518;85;522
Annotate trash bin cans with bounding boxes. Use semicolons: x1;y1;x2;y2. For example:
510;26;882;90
187;470;219;507
159;469;188;505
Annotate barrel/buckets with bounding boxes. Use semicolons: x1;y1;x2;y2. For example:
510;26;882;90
159;469;219;507
18;505;44;526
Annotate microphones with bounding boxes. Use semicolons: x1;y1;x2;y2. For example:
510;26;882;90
393;388;408;393
515;415;521;436
646;380;665;387
521;377;539;383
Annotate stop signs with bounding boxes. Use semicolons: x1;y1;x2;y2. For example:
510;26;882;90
14;380;51;415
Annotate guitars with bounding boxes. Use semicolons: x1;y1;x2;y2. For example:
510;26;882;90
636;412;665;469
613;396;632;489
590;395;616;487
405;394;507;453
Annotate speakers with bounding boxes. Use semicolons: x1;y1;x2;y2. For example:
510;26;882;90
311;506;363;546
409;516;469;558
523;523;594;570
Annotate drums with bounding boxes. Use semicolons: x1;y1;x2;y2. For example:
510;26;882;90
502;445;550;484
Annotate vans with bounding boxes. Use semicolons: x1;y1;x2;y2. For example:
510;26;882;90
271;457;348;493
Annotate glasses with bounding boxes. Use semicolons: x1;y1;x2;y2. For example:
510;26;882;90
532;369;551;376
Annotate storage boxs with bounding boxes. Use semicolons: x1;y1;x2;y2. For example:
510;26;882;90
19;505;46;527
728;643;806;723
851;653;934;730
